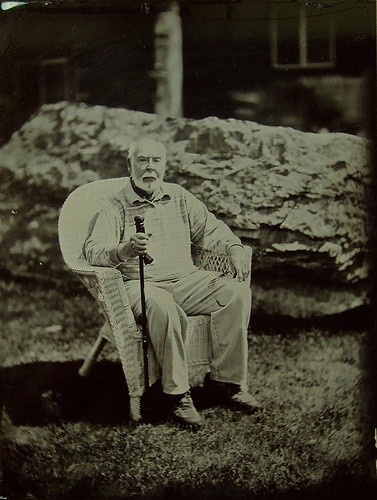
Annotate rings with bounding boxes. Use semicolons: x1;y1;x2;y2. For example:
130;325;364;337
130;241;136;249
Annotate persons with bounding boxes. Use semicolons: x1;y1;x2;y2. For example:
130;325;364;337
82;138;263;428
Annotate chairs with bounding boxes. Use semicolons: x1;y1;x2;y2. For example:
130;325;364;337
58;176;253;423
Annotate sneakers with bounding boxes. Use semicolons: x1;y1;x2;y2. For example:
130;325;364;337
167;390;204;431
223;386;262;414
